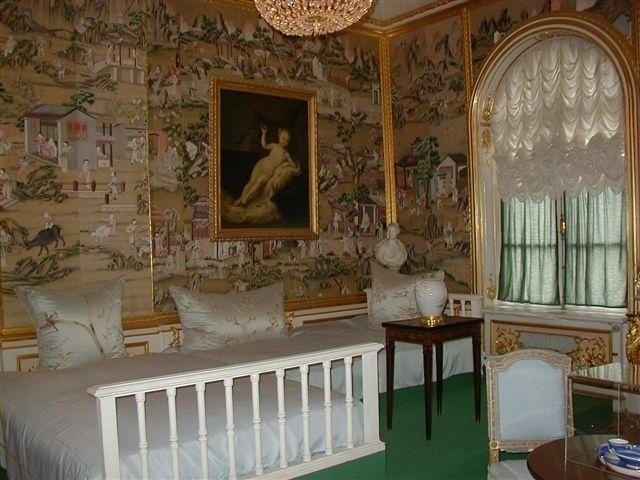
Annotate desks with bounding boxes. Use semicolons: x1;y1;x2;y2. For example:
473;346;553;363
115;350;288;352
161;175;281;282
572;362;640;438
527;433;638;479
381;314;484;440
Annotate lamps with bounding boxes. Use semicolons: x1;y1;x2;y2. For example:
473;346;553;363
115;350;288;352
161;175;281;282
255;0;372;38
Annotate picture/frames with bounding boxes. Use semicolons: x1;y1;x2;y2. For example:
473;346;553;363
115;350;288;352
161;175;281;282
205;74;320;241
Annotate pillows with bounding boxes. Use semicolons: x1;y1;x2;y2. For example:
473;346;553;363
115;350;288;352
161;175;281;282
370;260;445;324
166;281;287;350
14;273;129;370
364;284;422;330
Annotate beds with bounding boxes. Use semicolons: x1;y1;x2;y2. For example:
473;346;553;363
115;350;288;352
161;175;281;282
162;292;485;400
0;343;387;480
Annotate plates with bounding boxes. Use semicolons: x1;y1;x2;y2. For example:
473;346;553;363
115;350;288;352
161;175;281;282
596;443;640;478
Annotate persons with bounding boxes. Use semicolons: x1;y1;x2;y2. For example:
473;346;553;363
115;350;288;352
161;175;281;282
233;125;295;205
108;172;118;199
444;222;454;250
375;224;408;269
105;44;117;65
0;168;10;199
332;211;341;232
82;159;91;188
127;218;137;249
165;137;177;169
129;135;147;164
35;132;72;172
4;35;15;57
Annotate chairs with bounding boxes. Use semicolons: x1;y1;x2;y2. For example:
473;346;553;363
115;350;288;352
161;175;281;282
484;348;575;479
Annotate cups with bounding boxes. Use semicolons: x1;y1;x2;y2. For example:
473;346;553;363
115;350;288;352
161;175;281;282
608;439;633;454
